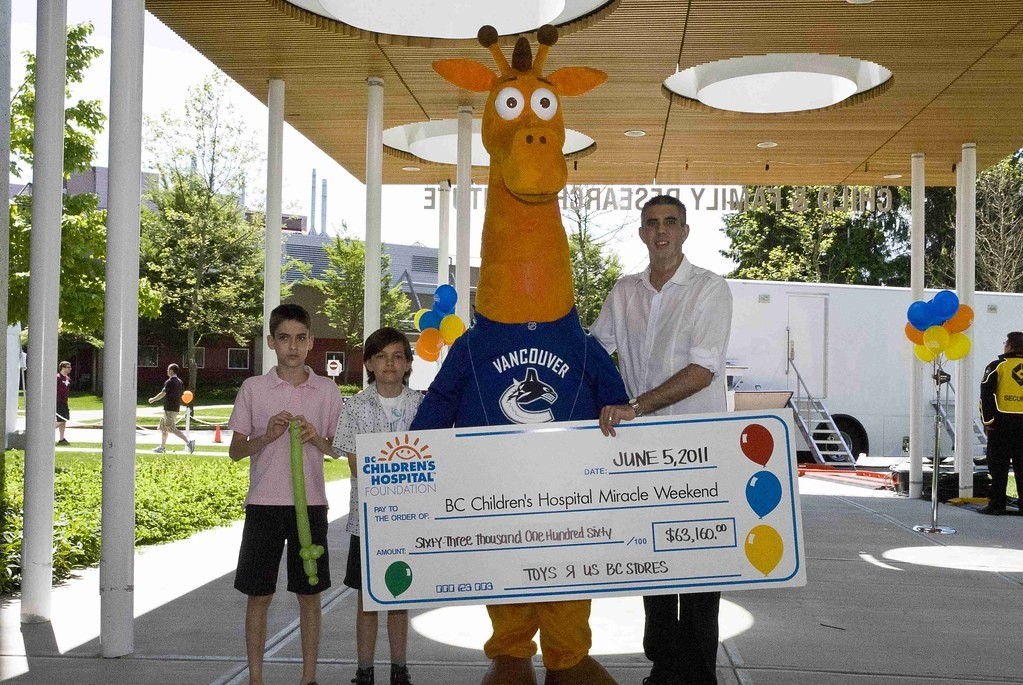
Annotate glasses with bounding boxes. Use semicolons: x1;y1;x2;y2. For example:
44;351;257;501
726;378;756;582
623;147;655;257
66;366;72;369
1002;342;1007;346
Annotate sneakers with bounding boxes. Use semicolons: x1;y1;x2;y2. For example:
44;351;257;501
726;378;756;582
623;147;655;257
187;439;196;454
58;438;70;445
151;446;166;454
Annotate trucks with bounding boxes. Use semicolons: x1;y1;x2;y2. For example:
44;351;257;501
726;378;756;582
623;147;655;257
724;279;1023;469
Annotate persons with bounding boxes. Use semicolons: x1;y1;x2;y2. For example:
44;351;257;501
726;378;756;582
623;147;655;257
586;195;732;685
56;361;71;445
148;364;195;454
227;304;344;685
344;327;424;685
976;332;1023;516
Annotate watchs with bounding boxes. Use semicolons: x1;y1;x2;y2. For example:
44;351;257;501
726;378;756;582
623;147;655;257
629;398;643;417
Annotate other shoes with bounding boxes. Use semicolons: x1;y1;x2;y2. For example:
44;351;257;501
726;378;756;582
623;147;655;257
640;666;681;685
389;663;415;685
975;503;1009;518
351;666;376;685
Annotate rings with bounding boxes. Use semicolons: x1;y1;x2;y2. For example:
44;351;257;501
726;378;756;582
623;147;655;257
609;417;613;421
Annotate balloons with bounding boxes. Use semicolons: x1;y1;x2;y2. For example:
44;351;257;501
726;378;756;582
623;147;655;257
905;289;975;366
414;285;465;363
182;391;193;404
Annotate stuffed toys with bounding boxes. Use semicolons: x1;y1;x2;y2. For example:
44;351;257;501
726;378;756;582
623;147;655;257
405;25;631;685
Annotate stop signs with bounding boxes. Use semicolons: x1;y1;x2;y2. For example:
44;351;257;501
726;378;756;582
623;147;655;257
328;360;339;376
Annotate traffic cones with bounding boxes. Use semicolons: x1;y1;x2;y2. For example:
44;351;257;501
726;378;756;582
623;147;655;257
213;424;223;443
